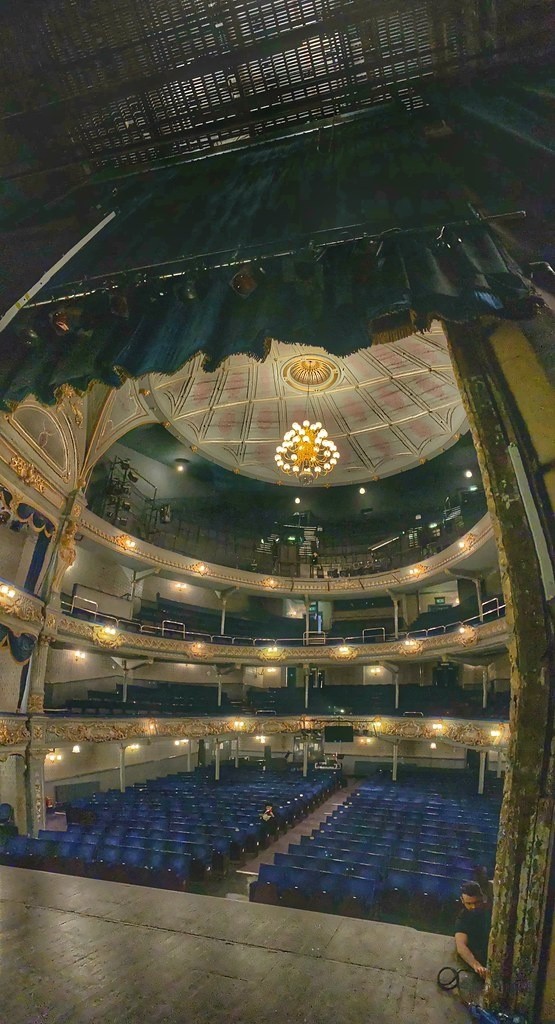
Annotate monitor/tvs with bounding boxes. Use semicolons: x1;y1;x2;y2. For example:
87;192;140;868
325;726;353;743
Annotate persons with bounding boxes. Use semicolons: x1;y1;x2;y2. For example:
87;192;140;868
261;802;278;840
472;866;494;899
454;880;493;980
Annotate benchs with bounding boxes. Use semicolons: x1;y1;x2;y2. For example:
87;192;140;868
1;760;506;937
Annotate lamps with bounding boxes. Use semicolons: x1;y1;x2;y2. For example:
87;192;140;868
72;746;81;753
429;741;438;749
275;360;342;487
